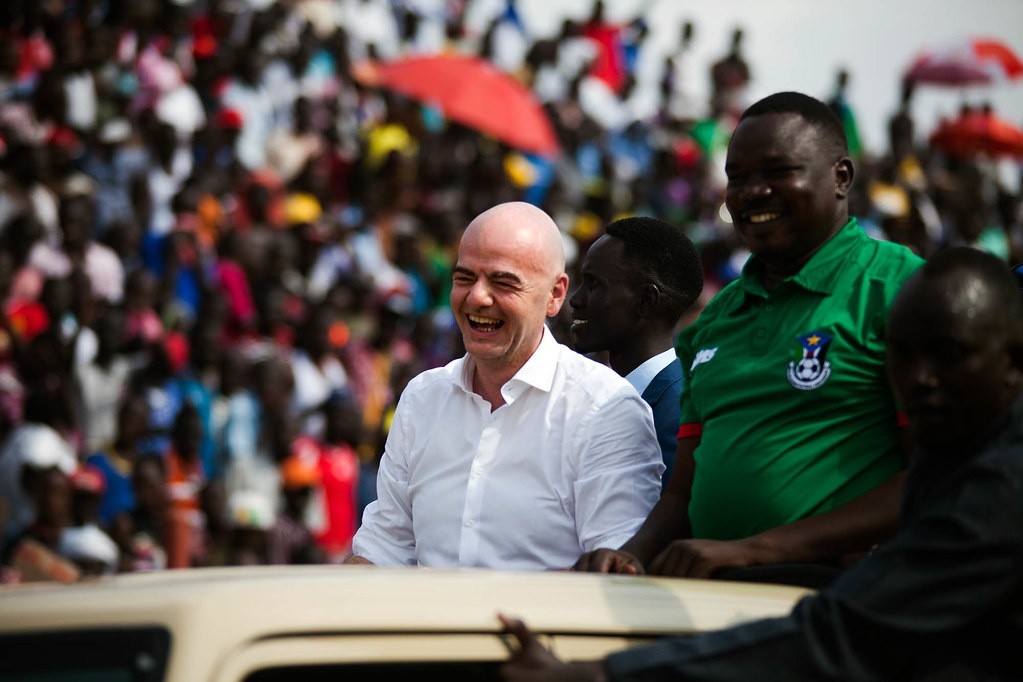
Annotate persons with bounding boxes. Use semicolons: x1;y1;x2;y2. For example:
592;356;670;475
444;0;1023;349
0;0;452;589
570;218;705;489
336;202;667;570
575;91;929;593
501;247;1023;682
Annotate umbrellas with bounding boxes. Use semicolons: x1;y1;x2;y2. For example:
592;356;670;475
904;37;1022;88
378;56;554;154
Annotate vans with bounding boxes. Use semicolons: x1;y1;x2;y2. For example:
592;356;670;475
0;565;817;682
